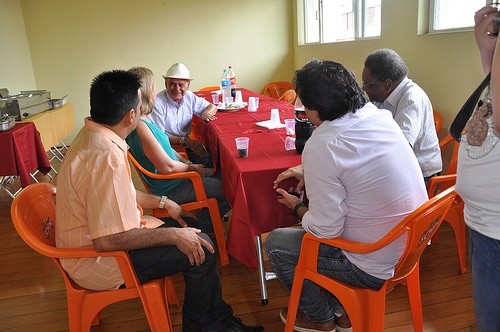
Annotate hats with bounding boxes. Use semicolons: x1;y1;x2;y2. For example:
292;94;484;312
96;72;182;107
161;63;194;81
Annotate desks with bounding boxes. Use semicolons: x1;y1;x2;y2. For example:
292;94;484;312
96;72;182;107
0;122;52;199
14;102;77;183
191;88;314;306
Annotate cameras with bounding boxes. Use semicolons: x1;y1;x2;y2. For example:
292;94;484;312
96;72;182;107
486;0;500;35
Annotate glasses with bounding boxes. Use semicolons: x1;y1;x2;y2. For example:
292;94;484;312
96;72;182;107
362;80;381;91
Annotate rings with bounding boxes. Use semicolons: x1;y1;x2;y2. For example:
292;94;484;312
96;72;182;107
486;30;498;37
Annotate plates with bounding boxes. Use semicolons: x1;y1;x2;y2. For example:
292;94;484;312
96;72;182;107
216;103;245;111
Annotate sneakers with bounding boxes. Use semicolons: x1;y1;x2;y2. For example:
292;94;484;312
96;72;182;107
334;313;352;332
222;317;264;332
279;307;336;332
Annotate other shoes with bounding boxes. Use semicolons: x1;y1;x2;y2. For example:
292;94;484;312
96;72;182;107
197;223;216;240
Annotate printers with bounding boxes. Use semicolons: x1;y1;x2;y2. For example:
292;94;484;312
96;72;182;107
0;87;53;121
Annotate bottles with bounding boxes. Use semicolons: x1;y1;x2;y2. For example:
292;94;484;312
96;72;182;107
294;97;317;155
228;65;237;102
220;68;231;107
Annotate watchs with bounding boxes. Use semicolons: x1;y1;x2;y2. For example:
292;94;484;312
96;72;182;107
179;137;185;145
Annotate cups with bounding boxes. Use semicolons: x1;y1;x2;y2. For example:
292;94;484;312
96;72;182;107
235;136;250;158
210;91;218;106
270;109;280;126
256;98;259;108
236;91;242;103
285;136;296;151
247;96;257;112
284;119;295;136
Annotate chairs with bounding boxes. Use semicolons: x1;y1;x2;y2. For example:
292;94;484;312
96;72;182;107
10;81;467;332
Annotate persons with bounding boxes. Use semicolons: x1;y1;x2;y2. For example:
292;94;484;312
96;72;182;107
55;69;264;332
454;1;500;332
124;65;233;227
361;48;443;199
151;62;219;178
263;59;431;332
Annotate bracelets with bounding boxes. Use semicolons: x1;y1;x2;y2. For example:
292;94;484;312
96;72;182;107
185;159;192;165
158;195;168;209
292;202;305;220
